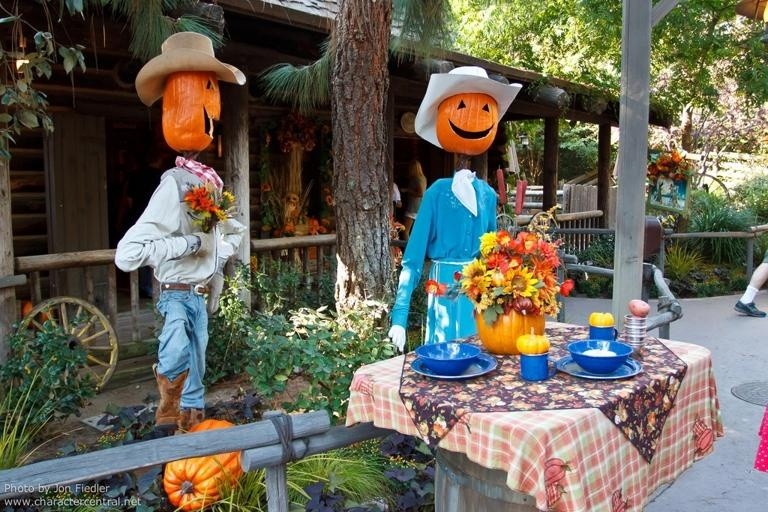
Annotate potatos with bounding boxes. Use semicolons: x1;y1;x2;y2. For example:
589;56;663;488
628;300;650;316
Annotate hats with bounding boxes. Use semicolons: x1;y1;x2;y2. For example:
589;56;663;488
414;66;524;150
135;31;246;107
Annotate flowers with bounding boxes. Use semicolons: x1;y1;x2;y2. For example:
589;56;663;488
456;222;573;317
647;146;695;186
257;106;336;242
178;180;241;235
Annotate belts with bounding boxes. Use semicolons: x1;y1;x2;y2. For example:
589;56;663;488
160;282;206;295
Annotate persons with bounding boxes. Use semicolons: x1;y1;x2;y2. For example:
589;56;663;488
398;157;427;244
112;32;247;433
391;182;402;216
733;250;768;318
387;64;524;354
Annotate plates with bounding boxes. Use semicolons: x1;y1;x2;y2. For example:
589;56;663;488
557;354;643;379
410;352;499;379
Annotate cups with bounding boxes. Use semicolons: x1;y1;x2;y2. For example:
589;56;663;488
589;325;618;341
520;352;558;381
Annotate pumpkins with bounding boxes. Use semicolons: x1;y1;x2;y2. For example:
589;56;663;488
165;421;244;508
516;332;552;354
588;312;615;327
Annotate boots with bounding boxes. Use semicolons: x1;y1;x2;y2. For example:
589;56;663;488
173;407;205;436
151;363;190;432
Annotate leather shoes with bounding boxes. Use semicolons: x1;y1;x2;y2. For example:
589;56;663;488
733;300;766;317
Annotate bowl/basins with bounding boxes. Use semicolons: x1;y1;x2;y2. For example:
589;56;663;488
416;341;481;376
567;339;634;374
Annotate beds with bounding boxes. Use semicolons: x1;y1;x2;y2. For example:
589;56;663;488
350;323;710;512
